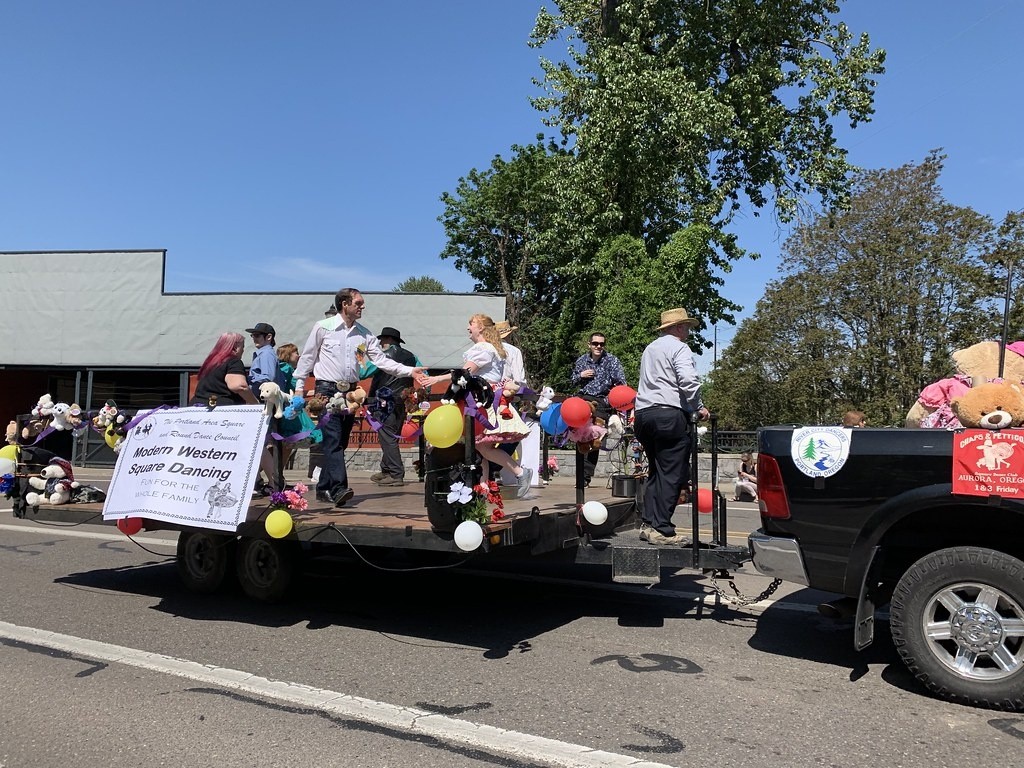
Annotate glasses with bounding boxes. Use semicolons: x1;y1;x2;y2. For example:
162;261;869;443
590;342;605;347
741;458;751;462
250;333;265;338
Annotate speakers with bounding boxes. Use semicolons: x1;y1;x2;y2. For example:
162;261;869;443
18;445;57;498
421;443;465;531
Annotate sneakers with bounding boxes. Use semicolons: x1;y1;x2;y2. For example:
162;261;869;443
514;465;533;498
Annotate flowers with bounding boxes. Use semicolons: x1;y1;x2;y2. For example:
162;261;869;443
266;481;309;513
447;480;506;523
697;426;709;445
539;456;560;481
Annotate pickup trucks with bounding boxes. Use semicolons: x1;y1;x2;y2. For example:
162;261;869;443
747;426;1024;713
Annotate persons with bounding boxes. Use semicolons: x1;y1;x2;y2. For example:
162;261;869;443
190;321;299;497
421;313;533;498
634;306;710;545
844;411;866;428
292;288;427;507
727;452;759;502
360;327;431;487
571;332;628;489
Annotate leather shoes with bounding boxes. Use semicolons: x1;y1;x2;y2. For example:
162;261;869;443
316;488;354;506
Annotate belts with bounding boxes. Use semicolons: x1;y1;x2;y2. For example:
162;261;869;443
653;403;673;408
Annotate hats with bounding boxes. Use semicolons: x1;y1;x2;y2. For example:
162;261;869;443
493;322;518;339
245;323;275;336
656;308;699;330
377;327;405;344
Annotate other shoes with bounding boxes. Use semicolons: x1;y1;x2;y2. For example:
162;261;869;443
639;522;688;545
370;472;404;486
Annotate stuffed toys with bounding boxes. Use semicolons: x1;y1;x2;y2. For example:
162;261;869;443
905;341;1024;430
258;366;555;420
577;400;625;452
0;394;130;506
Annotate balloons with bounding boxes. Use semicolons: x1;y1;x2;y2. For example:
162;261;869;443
561;397;590;426
264;509;292;539
582;501;609;525
697;487;714;513
454;520;483;551
539;401;567;436
423;403;464;449
608;386;637;411
118;517;142;535
